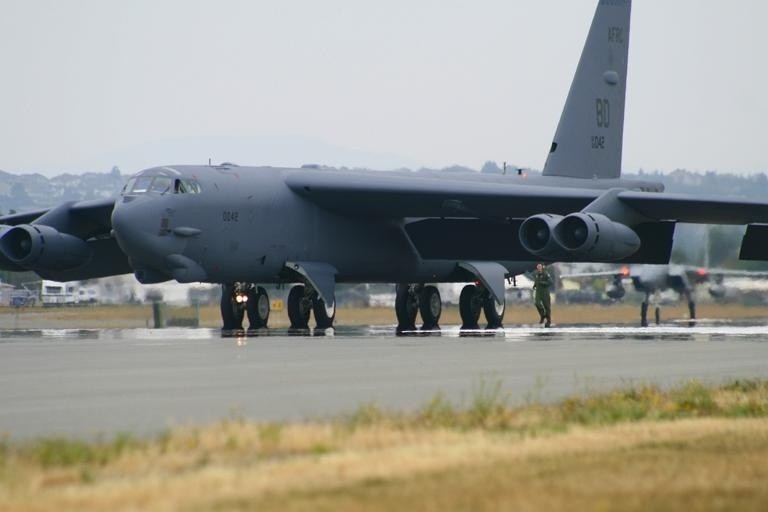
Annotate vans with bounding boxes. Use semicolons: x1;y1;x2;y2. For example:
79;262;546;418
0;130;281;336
74;287;98;303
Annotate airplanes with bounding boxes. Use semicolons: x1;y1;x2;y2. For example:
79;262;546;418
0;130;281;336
559;260;767;324
0;0;768;331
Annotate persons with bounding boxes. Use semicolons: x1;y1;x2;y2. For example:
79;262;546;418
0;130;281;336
533;263;553;326
